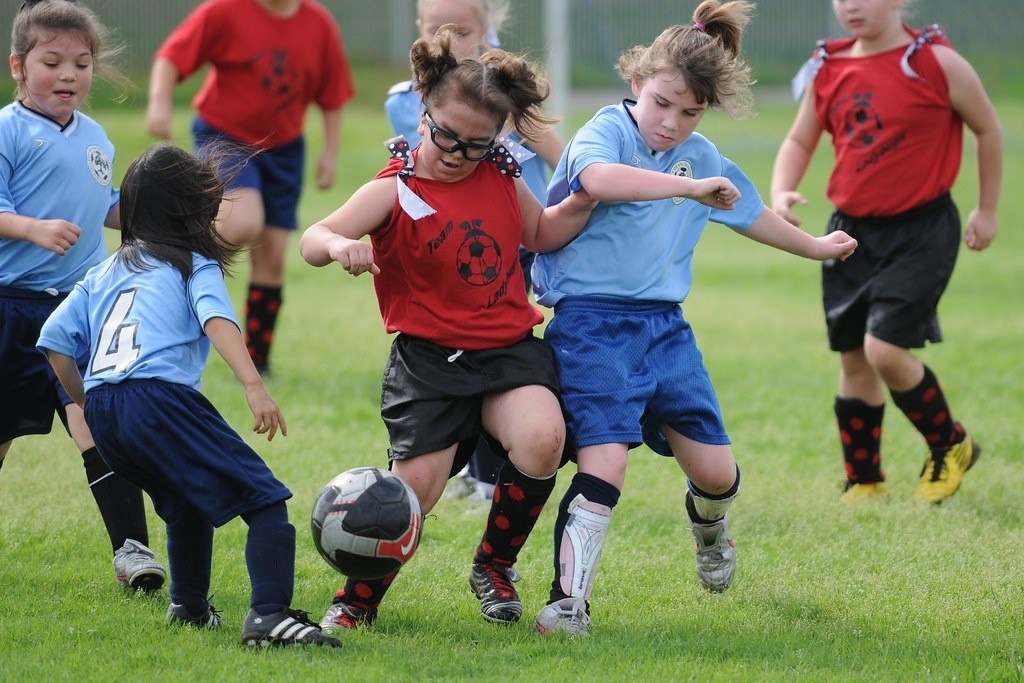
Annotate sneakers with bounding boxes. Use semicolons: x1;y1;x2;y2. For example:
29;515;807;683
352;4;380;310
242;607;343;650
912;438;982;506
469;562;523;624
840;480;885;499
690;519;737;594
320;602;378;630
166;594;222;631
535;596;592;639
116;538;167;591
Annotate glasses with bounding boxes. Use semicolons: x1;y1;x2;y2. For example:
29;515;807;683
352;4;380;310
422;112;495;165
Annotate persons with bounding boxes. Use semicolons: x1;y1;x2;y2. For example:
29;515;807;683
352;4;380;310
35;146;343;649
0;0;167;602
147;0;355;380
770;0;1005;504
385;0;568;505
528;0;858;638
298;31;600;635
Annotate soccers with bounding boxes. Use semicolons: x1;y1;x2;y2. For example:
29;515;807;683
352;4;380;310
309;466;424;580
